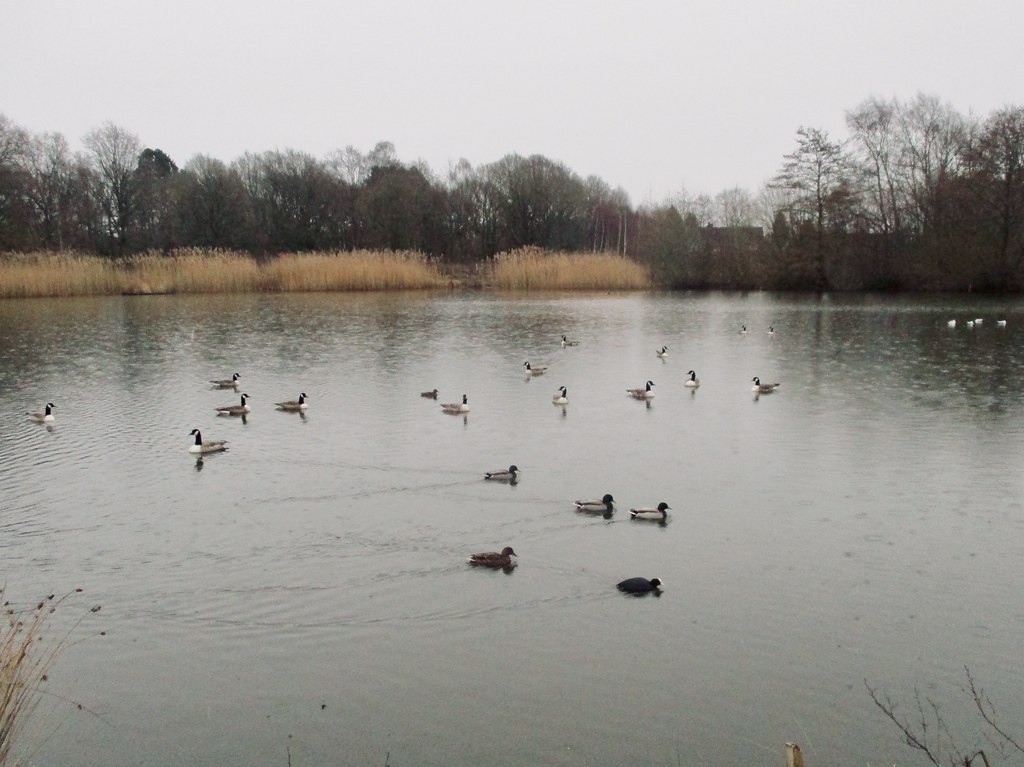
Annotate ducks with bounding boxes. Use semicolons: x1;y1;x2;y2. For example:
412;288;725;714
627;502;673;521
480;465;521;481
571;493;616;513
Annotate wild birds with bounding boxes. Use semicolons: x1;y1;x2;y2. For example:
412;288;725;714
552;385;569;404
439;393;470;412
626;381;656;397
418;388;438;397
212;393;251;414
616;576;664;592
208;372;242;386
465;546;519;567
684;370;699;386
655;346;668;356
188;429;231;452
736;324;748;334
751;377;781;391
561;335;582;349
22;402;56;421
947;318;1006;327
522;362;549;375
768;326;775;335
271;393;308;409
